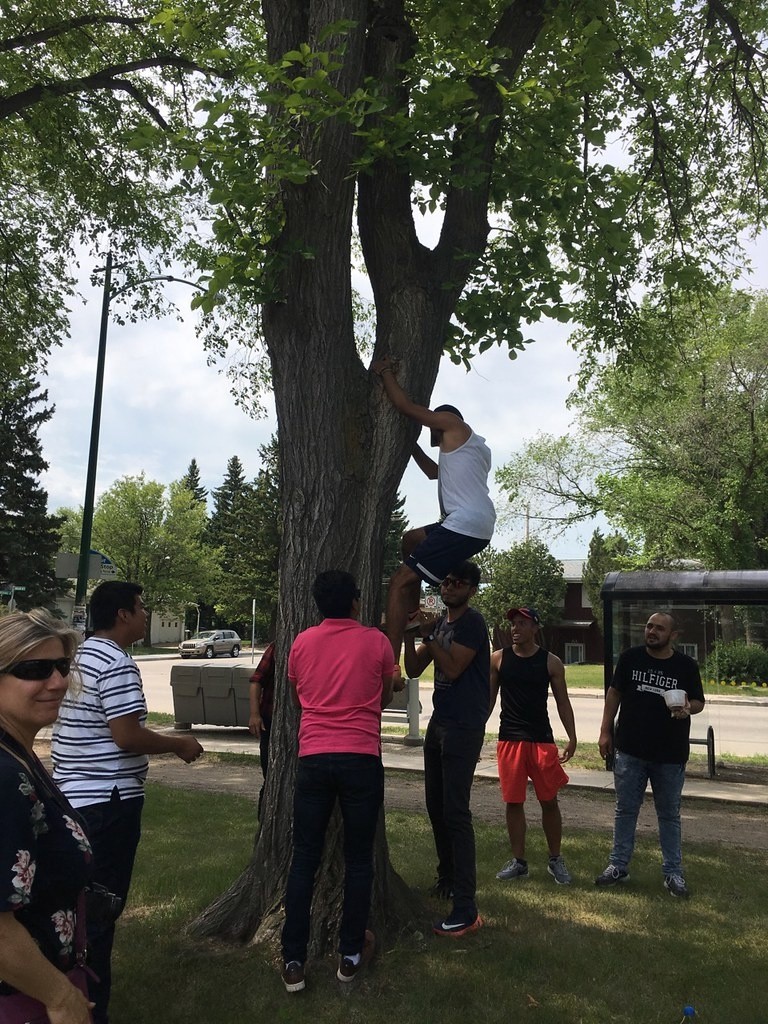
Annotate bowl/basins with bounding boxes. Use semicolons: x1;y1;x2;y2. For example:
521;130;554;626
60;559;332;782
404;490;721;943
664;689;686;711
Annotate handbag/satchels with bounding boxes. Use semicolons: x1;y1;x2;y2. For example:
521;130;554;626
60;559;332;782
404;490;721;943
0;966;99;1024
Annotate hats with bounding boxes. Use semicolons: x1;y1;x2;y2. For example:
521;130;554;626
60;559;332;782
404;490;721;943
508;608;540;624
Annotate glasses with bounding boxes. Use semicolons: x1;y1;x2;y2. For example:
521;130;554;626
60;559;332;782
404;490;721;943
0;657;71;680
442;578;472;589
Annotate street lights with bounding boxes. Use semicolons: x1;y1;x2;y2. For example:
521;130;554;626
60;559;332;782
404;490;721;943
72;250;231;653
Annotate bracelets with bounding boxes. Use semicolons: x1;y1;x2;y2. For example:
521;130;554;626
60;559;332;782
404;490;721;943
381;367;392;376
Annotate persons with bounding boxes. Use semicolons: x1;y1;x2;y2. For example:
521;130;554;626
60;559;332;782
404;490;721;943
0;608;96;1024
593;613;706;897
477;608;577;885
51;581;203;1024
404;561;491;936
248;641;275;821
281;571;406;992
371;354;496;679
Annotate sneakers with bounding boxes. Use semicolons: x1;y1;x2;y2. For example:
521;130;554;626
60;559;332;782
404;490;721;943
494;858;530;880
337;930;374;982
430;881;453;901
433;912;483;935
595;864;630;887
547;855;572;885
664;873;688;897
281;962;305;991
393;665;401;678
376;609;423;632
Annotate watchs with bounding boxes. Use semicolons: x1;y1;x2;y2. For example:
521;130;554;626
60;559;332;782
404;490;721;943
423;634;435;642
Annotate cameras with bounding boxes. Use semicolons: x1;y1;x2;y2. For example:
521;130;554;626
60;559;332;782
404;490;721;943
81;882;124;931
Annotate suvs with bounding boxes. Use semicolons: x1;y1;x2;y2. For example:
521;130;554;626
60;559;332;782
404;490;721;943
178;629;243;660
